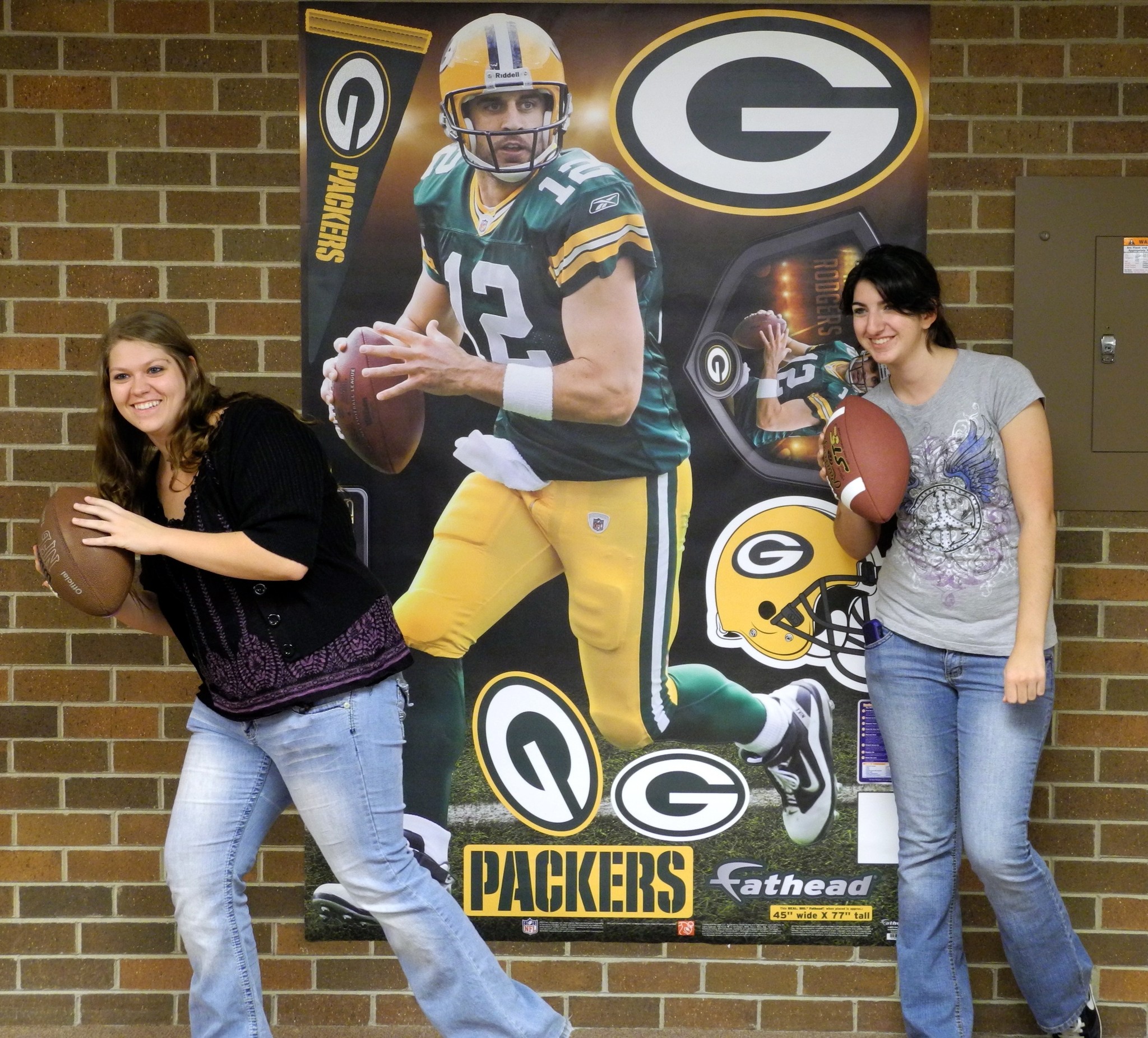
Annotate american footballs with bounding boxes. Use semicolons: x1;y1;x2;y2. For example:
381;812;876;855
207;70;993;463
823;394;911;524
330;326;425;475
37;486;135;617
731;313;787;349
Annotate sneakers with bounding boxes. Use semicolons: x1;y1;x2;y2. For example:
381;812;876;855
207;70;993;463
735;678;834;845
313;828;454;928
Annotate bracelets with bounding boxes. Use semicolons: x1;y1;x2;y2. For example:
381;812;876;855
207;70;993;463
756;378;783;398
503;363;553;421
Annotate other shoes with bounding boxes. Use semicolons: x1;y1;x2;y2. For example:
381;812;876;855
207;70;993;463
1050;979;1101;1038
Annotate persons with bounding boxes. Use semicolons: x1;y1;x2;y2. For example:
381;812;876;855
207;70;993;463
728;309;891;448
33;309;574;1038
816;244;1102;1038
309;10;833;932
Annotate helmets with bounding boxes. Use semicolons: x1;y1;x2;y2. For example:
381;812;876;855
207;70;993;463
437;14;569;183
844;350;892;397
